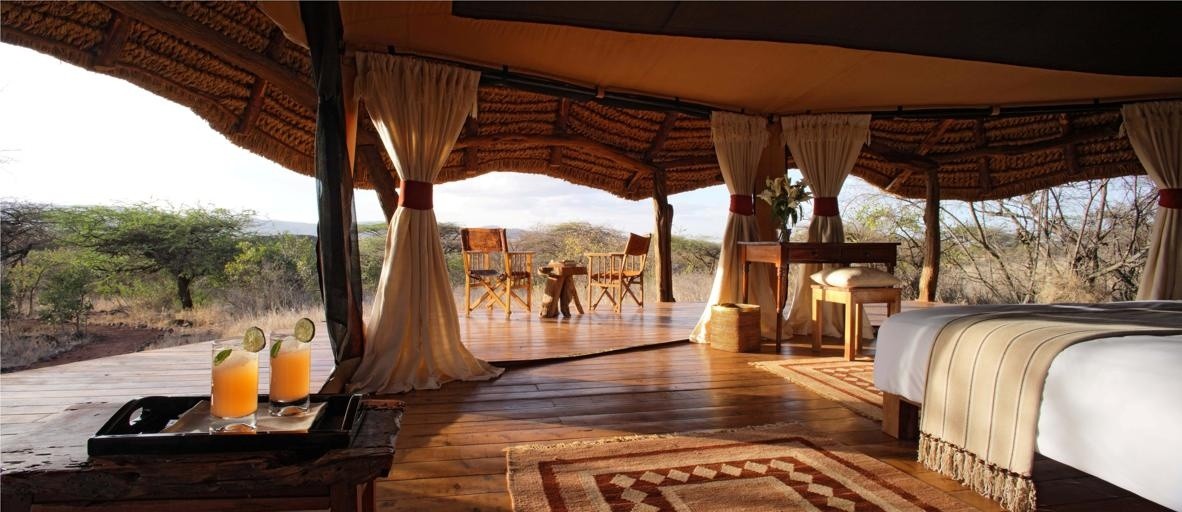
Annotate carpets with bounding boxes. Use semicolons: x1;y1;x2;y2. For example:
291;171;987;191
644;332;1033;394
748;357;883;429
502;419;984;512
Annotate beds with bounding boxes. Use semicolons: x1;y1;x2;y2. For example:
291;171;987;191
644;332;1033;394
873;300;1182;512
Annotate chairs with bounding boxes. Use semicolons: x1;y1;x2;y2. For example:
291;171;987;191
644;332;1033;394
459;228;536;318
587;233;651;313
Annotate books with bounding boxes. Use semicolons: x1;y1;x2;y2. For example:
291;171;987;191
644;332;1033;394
558;260;576;269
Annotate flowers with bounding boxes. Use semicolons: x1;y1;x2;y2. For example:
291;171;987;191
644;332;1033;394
756;173;816;229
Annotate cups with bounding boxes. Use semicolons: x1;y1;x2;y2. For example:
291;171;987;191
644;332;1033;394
267;326;311;417
208;334;260;433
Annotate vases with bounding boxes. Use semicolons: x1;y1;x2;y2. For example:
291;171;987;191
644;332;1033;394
776;216;791;242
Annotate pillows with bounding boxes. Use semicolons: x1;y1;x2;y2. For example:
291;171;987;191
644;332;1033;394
810;267;899;288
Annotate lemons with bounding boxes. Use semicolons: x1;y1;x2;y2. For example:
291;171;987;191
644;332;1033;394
243;326;265;352
294;318;315;343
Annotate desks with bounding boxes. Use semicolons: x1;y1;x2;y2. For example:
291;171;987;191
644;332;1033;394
0;398;408;512
537;267;587;318
737;241;901;353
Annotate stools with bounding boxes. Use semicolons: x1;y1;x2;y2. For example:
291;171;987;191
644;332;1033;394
810;285;903;361
